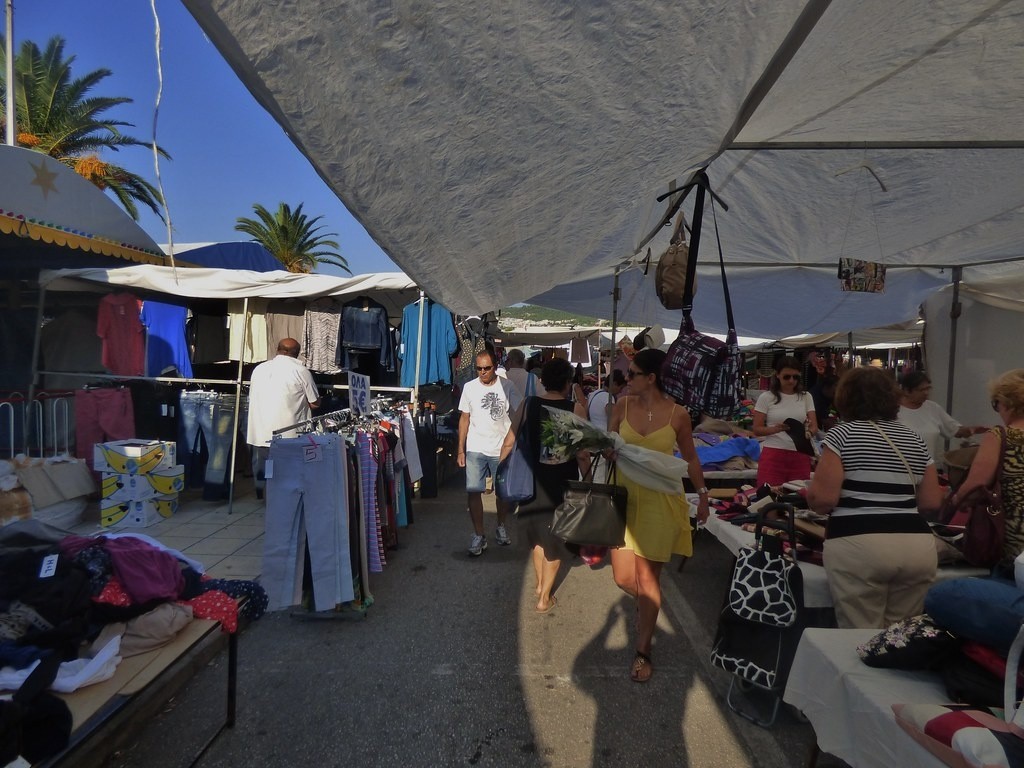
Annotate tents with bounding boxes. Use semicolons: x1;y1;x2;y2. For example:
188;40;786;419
177;0;1024;450
24;264;425;429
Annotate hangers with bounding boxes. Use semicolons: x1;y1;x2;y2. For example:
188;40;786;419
616;254;649;277
456;315;484;327
641;247;651;276
670;212;692;245
82;375;250;395
294;391;434;441
656;165;729;213
835;141;887;193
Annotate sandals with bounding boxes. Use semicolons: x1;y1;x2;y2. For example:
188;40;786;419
631;650;655;682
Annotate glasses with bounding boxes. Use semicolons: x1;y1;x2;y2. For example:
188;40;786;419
505;358;509;362
474;364;494;370
991;398;1001;412
627;367;643;378
782;374;801;380
912;385;932;392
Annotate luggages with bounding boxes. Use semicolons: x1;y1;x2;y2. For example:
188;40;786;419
710;502;811;729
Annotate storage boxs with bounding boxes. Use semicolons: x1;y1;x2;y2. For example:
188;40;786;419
100;492;178;527
102;465;184;501
93;439;176;475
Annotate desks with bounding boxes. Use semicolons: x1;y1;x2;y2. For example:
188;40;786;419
35;593;249;768
782;628;960;768
682;441;761;478
678;493;991;605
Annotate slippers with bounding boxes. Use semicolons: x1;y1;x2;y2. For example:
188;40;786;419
535;594;556;613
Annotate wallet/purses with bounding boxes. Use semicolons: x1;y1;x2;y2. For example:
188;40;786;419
688;480;830;565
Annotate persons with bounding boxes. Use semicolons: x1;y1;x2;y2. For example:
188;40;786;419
245;337;322;505
457;348;1024;682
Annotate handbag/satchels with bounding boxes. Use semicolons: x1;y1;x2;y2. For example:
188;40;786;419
658;315;746;420
579;546;607;567
550;451;629;550
495;399;534;501
511;372;536;437
963;424;1006;568
653;211;697;311
857;552;1024;768
617;325;665;361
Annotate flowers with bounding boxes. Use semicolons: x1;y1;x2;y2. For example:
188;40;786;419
541;410;681;493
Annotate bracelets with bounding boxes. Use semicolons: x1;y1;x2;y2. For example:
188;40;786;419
697;486;708;495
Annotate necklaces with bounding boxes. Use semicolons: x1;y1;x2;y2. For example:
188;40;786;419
638;394;662;421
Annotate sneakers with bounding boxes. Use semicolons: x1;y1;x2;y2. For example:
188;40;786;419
495;520;511;545
465;533;488;556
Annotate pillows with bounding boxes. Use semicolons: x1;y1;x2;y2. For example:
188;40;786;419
857;550;1024;768
